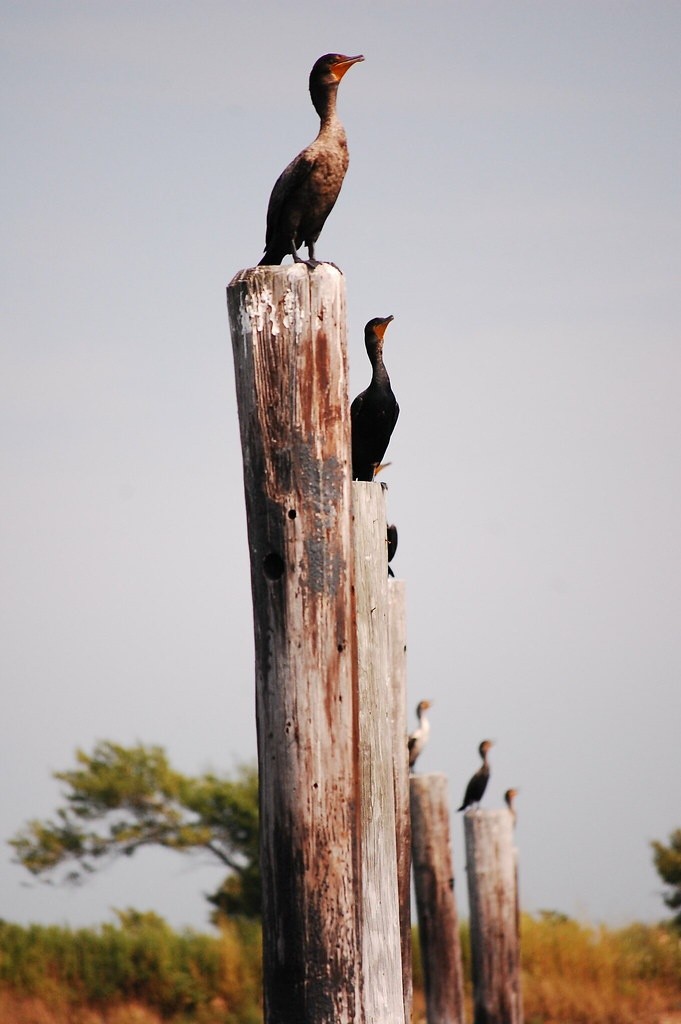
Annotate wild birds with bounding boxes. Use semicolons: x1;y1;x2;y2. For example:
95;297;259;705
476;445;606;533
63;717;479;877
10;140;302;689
388;523;398;580
258;50;367;276
350;314;400;491
407;697;434;774
457;740;499;813
503;787;520;831
